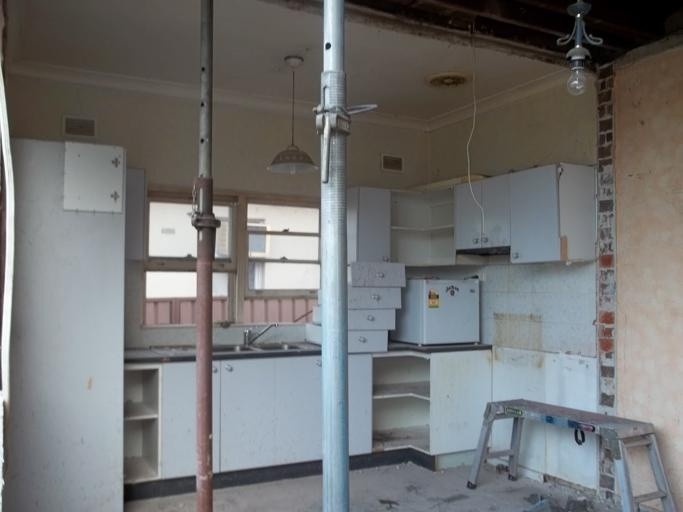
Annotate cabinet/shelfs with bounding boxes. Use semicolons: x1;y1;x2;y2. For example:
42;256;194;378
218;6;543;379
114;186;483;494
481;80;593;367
123;348;493;486
346;186;391;265
510;163;597;264
455;172;511;250
391;189;428;267
428;184;485;267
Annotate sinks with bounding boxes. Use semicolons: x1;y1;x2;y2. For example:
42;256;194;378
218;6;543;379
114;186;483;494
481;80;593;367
149;345;252;355
252;343;305;351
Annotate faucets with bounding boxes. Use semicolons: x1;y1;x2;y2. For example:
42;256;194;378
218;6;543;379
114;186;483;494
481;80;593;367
244;323;278;345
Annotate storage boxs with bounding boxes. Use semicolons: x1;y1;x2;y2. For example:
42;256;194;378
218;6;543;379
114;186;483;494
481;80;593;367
305;262;406;353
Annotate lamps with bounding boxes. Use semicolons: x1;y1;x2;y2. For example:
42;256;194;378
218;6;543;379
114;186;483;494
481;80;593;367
267;56;320;176
556;0;604;97
